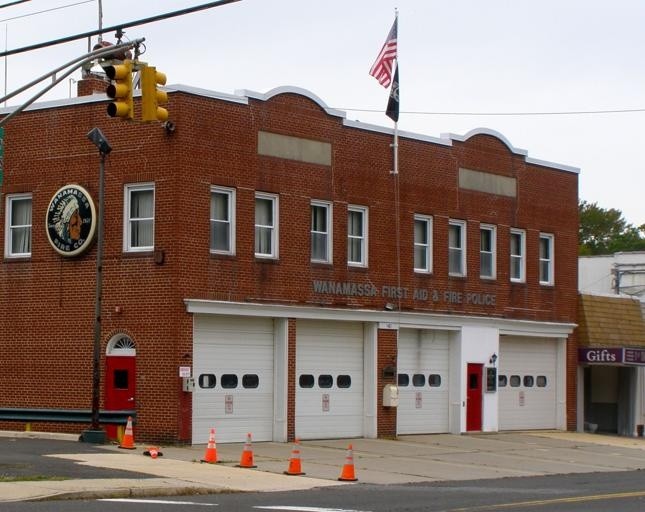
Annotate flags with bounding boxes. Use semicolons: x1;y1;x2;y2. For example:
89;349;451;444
369;17;399;123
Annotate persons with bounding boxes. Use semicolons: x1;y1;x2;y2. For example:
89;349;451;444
53;195;82;244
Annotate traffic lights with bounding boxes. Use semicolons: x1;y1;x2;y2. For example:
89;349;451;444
103;58;134;123
140;65;170;124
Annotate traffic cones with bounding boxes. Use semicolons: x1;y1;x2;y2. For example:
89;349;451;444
144;447;164;459
338;442;359;481
118;416;137;450
233;432;258;468
200;426;223;464
283;436;307;475
